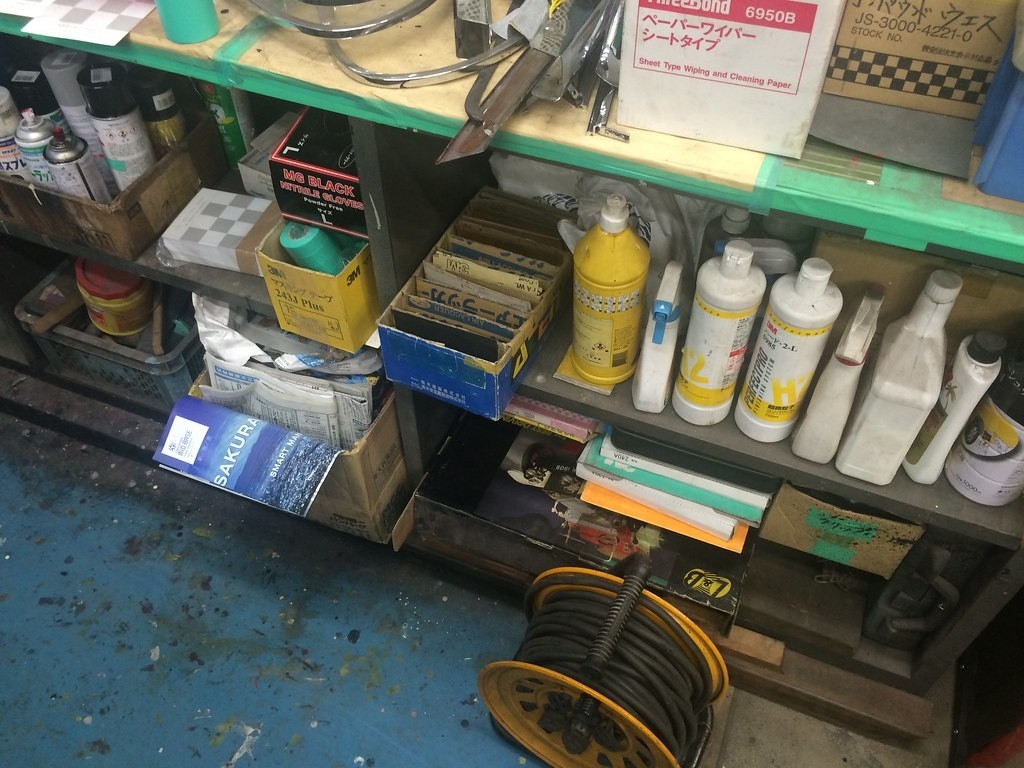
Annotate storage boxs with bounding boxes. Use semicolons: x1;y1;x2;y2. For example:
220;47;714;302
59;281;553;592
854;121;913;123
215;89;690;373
15;259;206;409
811;227;1024;354
616;0;846;160
822;0;1017;120
152;367;455;544
268;106;370;240
238;147;276;202
376;186;579;421
758;480;927;579
255;218;379;353
0;107;229;261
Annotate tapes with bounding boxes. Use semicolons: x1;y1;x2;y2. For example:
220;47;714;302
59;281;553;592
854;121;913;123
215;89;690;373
281;220;345;275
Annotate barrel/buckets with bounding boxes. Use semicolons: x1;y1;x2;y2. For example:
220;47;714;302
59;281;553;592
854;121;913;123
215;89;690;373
74;256;163;336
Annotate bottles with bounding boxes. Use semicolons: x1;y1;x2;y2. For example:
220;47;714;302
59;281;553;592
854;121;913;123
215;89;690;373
198;79;247;170
0;48;189;205
734;257;843;443
156;0;220;45
703;204;799;379
573;194;651;385
902;329;1007;485
672;241;766;426
943;355;1024;505
837;271;962;485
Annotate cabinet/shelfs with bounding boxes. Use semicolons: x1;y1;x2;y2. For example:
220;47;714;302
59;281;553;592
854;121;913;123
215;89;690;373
2;0;1023;698
